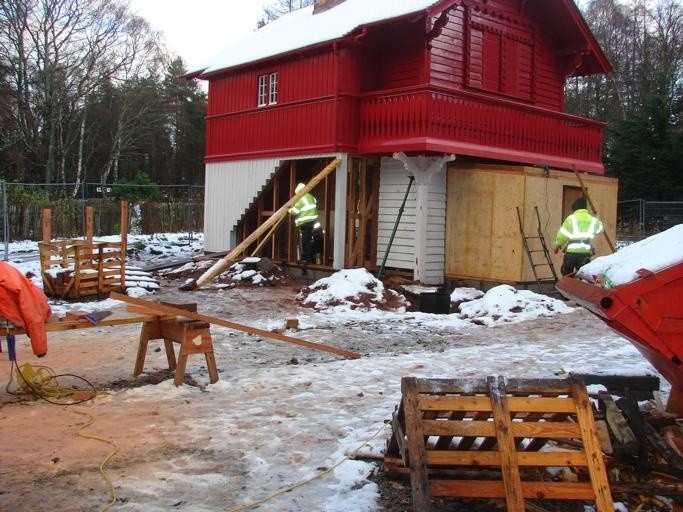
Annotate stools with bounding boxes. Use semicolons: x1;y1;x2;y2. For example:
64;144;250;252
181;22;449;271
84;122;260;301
133;314;219;387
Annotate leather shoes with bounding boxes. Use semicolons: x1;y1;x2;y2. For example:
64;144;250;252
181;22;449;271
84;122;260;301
561;296;570;301
297;260;309;266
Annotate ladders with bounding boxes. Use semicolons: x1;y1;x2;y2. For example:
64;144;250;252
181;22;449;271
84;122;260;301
515;206;563;298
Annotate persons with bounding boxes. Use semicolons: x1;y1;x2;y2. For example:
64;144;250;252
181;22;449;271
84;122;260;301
288;183;319;263
552;197;604;277
